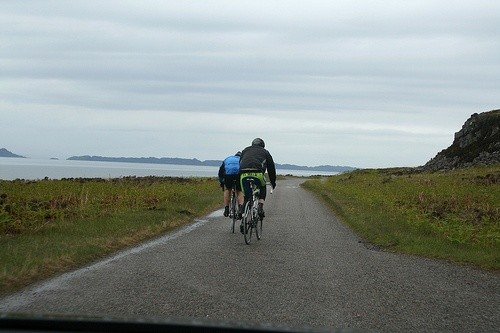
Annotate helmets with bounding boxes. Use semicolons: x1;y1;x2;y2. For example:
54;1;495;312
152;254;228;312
252;138;265;148
235;152;242;157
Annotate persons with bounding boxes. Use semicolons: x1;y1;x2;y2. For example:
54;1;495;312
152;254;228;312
218;151;245;219
239;138;277;234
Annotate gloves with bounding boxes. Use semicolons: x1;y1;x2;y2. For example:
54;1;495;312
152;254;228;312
220;182;224;188
270;182;276;189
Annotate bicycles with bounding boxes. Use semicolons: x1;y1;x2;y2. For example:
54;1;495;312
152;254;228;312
220;181;237;234
238;178;273;244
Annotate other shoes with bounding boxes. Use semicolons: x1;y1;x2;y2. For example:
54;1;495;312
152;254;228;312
258;207;264;218
240;223;248;235
224;207;229;217
